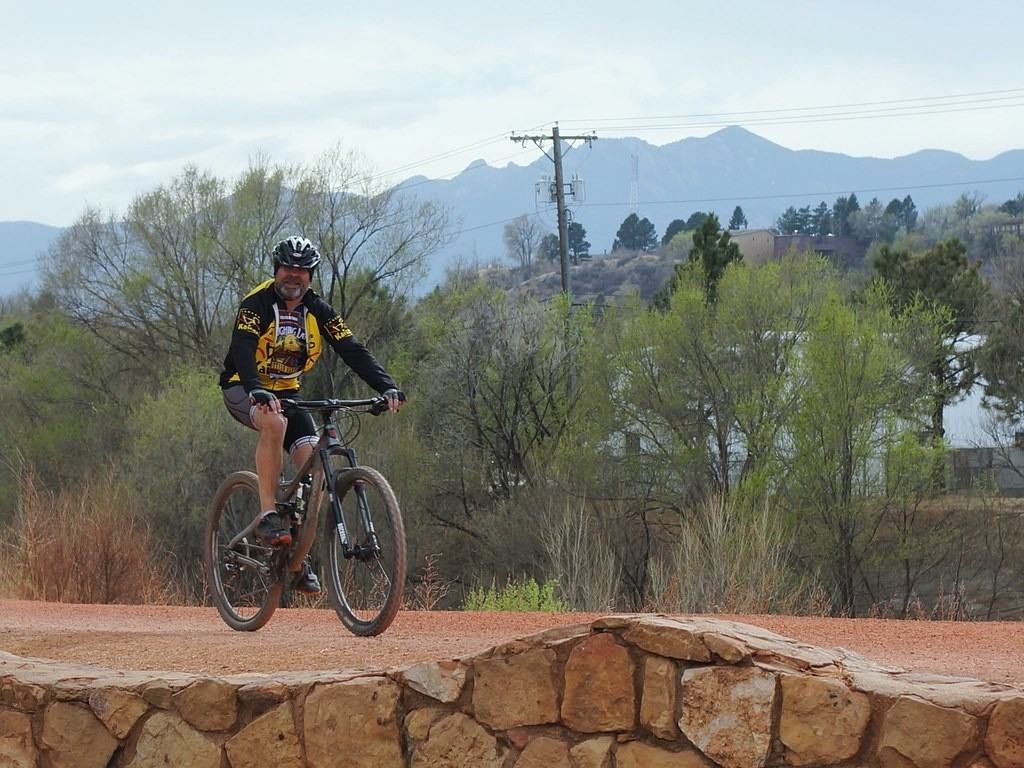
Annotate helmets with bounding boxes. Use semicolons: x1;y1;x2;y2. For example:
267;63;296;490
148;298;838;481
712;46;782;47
272;236;321;269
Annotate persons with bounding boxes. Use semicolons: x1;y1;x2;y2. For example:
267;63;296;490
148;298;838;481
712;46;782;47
218;235;407;594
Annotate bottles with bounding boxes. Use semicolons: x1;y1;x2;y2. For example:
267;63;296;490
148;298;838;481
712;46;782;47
294;472;312;519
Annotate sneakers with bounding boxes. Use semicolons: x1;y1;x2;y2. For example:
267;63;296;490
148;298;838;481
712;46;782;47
295;555;321;596
254;512;292;544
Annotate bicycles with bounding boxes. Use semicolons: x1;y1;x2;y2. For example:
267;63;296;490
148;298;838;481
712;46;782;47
203;397;408;638
